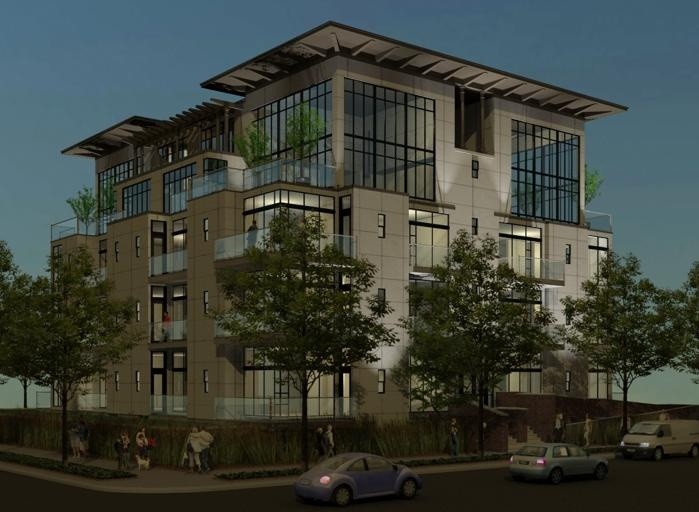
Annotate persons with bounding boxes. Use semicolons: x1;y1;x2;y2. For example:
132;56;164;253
160;310;171;342
658;408;670;423
554;413;563;443
246;220;258;249
619;413;631;436
313;427;323;460
323;424;335;461
68;420;90;459
186;426;214;473
113;426;148;472
584;413;593;448
448;417;460;457
